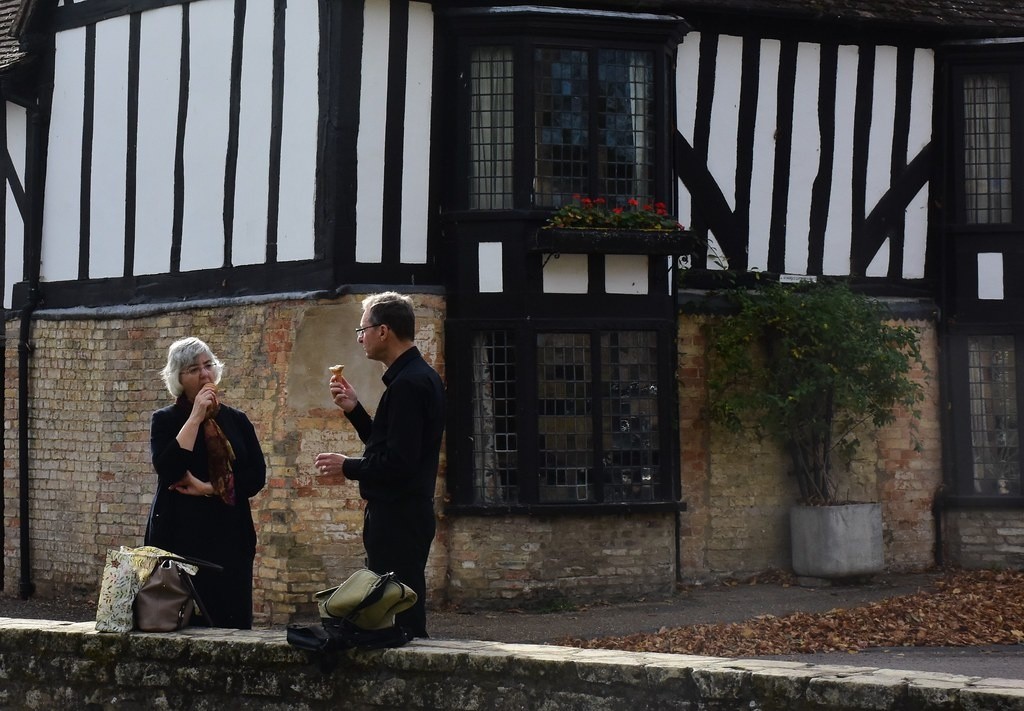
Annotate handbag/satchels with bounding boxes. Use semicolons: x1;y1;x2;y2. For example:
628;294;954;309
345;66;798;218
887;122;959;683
286;570;419;648
94;546;224;633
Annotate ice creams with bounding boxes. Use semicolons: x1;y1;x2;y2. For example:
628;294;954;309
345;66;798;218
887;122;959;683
202;383;218;395
329;364;344;384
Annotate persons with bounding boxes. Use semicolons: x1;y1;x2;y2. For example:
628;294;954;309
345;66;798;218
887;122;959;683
314;292;449;638
143;336;267;629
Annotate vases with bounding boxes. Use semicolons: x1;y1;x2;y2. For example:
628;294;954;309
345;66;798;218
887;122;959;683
535;226;697;253
789;502;888;576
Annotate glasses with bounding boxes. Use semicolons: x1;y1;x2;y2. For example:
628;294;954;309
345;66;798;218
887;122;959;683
181;362;217;376
355;323;390;337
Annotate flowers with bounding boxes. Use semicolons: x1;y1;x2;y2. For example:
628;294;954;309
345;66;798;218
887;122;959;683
542;193;686;230
664;232;937;504
